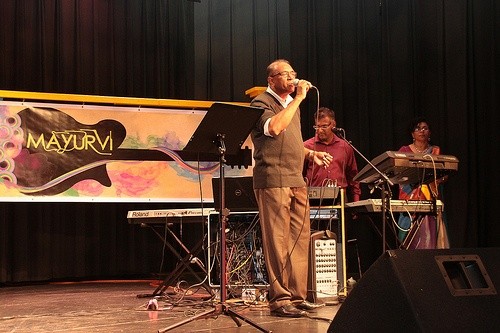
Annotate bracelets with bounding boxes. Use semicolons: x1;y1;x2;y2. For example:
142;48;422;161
307;150;313;161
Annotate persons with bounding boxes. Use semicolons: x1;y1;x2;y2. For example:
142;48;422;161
249;58;326;318
303;108;362;274
396;120;443;252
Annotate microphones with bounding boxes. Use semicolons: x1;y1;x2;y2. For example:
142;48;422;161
332;127;343;133
293;79;314;90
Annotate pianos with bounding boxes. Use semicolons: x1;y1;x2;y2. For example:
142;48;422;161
126;208;221;300
353;151;459;257
342;198;444;255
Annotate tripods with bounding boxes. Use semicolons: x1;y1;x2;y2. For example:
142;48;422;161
158;102;275;333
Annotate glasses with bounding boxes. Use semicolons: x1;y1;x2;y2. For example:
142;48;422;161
271;71;297;77
313;122;331;129
414;126;429;132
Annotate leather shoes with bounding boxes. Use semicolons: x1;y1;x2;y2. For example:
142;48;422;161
295;300;325;311
270;304;310;316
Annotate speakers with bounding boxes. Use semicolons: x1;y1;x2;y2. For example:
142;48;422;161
328;248;500;333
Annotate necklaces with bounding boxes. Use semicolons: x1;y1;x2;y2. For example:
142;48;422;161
413;144;430;154
